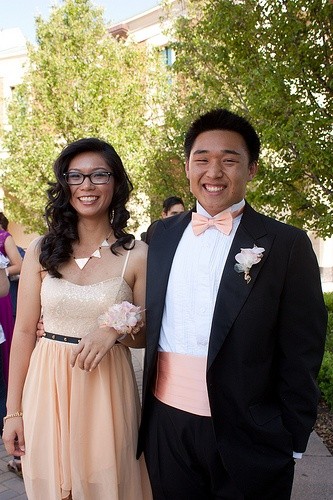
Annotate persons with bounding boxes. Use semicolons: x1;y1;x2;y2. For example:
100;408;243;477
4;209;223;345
163;197;184;218
0;212;23;476
36;108;328;500
2;139;152;500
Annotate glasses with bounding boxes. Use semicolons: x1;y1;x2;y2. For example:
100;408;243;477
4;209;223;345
63;171;112;185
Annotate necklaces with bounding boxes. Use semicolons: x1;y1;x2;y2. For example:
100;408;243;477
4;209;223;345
71;230;113;270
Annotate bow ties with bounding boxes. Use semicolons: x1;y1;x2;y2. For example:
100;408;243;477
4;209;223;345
192;211;233;236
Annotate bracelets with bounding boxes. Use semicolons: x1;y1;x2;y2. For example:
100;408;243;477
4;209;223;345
4;413;23;420
101;305;142;341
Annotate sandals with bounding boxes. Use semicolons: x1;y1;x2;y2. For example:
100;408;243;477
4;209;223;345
7;457;23;478
